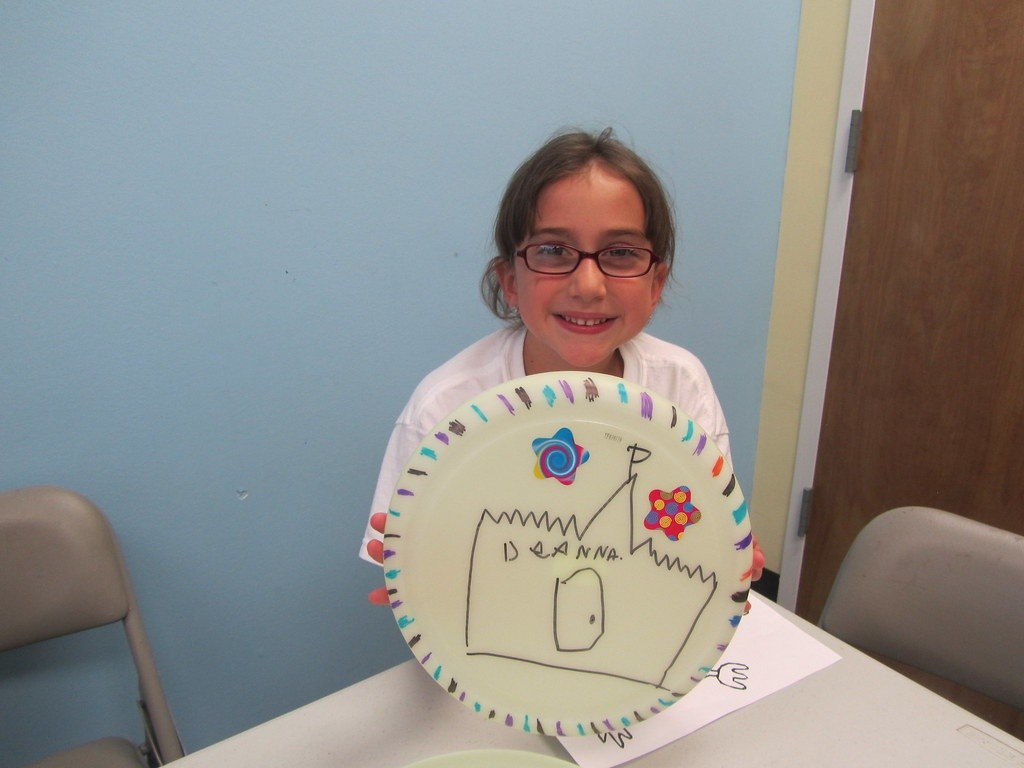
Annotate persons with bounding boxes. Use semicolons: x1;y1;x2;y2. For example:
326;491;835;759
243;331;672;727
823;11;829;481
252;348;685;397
356;128;765;610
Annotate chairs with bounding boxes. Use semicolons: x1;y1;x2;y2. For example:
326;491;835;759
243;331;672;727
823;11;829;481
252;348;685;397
0;484;187;768
817;506;1023;714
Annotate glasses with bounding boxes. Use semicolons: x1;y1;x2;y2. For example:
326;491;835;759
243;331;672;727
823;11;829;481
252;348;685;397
514;240;661;278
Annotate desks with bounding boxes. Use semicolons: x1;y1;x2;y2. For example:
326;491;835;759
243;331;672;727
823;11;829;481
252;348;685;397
159;586;1024;768
